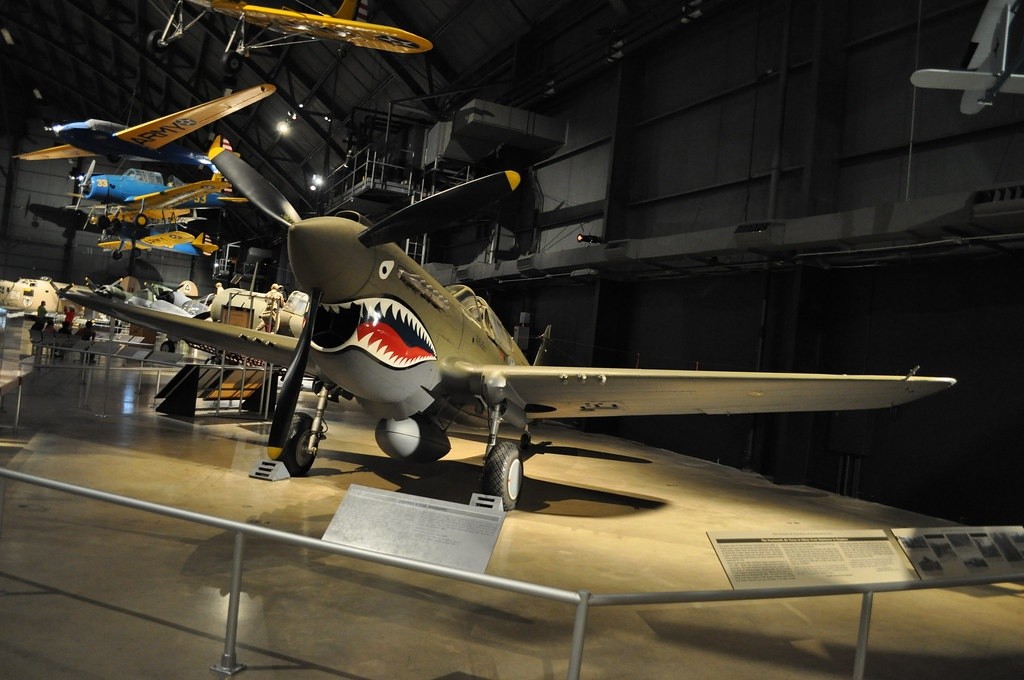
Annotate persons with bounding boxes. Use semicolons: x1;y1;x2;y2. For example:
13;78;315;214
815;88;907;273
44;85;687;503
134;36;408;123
75;321;96;363
114;319;122;333
32;301;76;356
216;282;223;295
256;283;287;335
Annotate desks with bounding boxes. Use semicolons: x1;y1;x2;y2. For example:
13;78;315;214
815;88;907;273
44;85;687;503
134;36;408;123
0;370;31;436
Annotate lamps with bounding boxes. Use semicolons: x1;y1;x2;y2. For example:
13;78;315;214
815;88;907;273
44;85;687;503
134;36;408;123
680;0;704;25
543;72;561;99
733;222;785;246
577;233;603;244
608;37;626;64
517;254;534;272
455;266;474;281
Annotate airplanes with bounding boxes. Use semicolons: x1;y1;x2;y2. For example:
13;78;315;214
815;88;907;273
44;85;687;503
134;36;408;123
0;0;1024;512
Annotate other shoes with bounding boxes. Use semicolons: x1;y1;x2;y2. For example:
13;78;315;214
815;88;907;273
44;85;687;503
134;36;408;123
89;360;97;364
